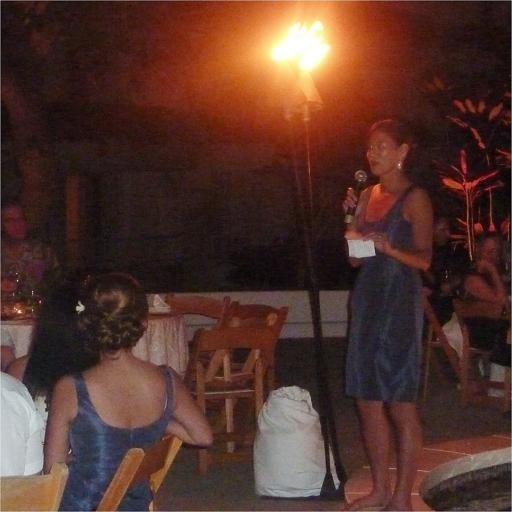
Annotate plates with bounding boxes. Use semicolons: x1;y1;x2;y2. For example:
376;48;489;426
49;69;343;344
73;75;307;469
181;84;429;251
148;306;171;315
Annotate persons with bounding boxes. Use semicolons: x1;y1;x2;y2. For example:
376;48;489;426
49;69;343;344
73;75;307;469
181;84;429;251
462;225;511;371
336;110;436;510
0;370;46;477
418;210;466;362
42;268;216;512
1;196;61;297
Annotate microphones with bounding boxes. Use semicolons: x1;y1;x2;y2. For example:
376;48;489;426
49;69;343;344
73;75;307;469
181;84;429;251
343;169;368;223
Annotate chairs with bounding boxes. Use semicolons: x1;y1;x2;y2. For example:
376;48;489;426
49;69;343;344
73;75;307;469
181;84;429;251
454;299;511;414
162;292;230;368
93;433;185;510
184;323;281;473
192;300;287;396
0;458;72;511
421;295;461;402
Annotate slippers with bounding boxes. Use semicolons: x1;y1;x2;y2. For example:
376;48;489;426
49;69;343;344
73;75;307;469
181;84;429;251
343;497;404;511
